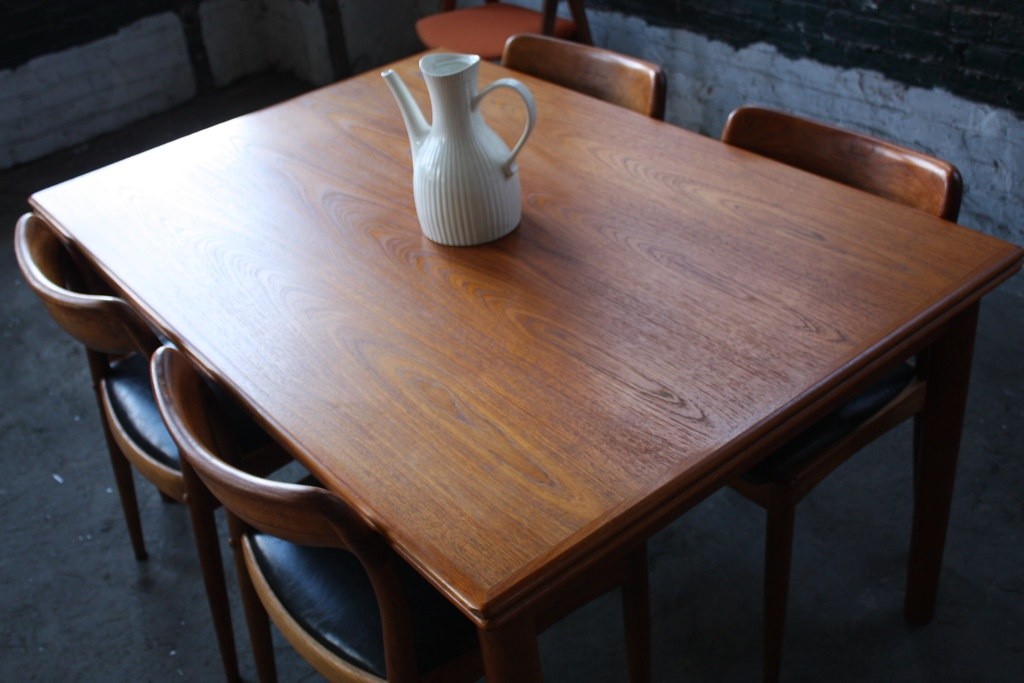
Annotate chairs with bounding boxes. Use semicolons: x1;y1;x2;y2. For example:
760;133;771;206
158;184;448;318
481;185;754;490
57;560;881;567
416;0;594;63
501;31;669;123
15;212;268;683
720;105;964;683
149;342;654;683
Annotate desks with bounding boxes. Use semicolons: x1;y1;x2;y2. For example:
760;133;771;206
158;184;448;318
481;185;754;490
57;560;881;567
27;48;1022;683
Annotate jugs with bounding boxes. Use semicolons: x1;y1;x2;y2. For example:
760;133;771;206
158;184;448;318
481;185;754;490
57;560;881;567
381;51;536;246
